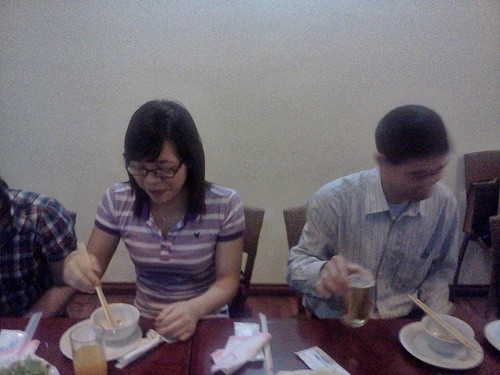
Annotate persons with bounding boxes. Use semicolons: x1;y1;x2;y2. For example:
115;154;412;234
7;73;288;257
0;174;79;318
284;104;461;320
63;99;246;341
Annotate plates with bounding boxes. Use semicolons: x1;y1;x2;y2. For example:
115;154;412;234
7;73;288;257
59;319;143;361
398;322;485;369
484;320;500;351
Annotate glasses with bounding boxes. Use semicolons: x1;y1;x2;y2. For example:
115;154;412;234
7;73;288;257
134;160;183;178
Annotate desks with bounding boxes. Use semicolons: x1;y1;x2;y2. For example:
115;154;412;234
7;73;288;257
0;316;192;375
190;316;500;375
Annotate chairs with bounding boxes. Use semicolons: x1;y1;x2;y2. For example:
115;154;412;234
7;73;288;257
227;208;264;318
449;150;500;319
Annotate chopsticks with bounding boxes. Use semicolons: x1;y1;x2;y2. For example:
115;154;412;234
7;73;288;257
259;312;273;375
76;240;116;328
411;296;477;350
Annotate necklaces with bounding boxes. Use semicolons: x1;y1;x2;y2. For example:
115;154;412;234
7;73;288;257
156;207;182;228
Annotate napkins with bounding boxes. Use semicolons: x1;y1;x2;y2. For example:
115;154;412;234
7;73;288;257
1;330;41;359
210;332;272;375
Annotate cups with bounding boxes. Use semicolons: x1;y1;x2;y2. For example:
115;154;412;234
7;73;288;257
340;268;376;328
68;324;108;375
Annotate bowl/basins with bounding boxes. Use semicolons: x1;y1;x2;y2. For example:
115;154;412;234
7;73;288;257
89;302;140;347
422;314;474;356
